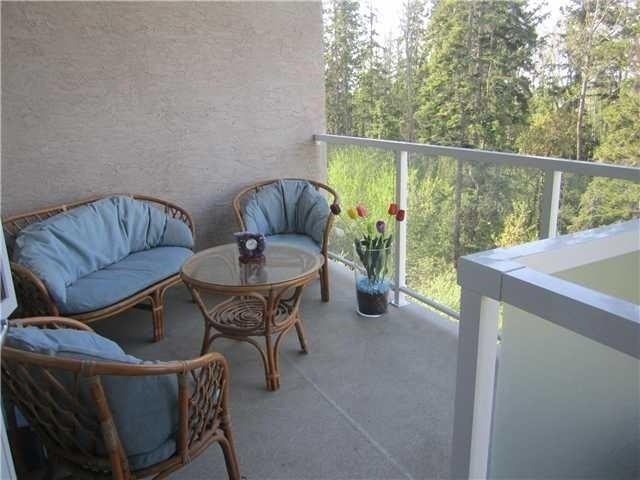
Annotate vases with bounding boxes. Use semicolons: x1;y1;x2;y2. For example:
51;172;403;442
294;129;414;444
351;239;394;318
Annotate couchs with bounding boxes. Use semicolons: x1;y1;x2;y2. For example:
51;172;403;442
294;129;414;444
2;191;196;344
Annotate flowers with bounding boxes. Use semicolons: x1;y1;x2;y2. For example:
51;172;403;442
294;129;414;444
330;203;405;287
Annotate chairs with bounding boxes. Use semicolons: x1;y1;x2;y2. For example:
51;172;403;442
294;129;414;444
231;177;340;302
0;316;242;479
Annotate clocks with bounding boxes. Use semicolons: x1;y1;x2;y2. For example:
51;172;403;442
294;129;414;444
233;231;266;265
